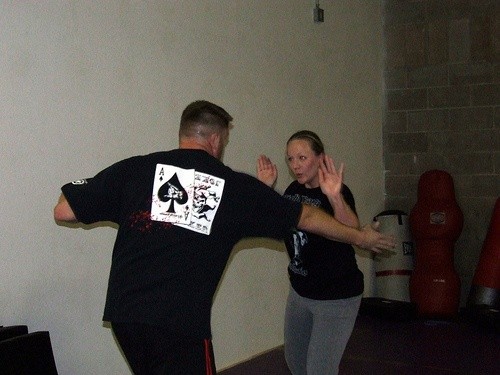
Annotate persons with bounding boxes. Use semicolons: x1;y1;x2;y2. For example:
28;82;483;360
255;130;366;374
53;99;398;375
